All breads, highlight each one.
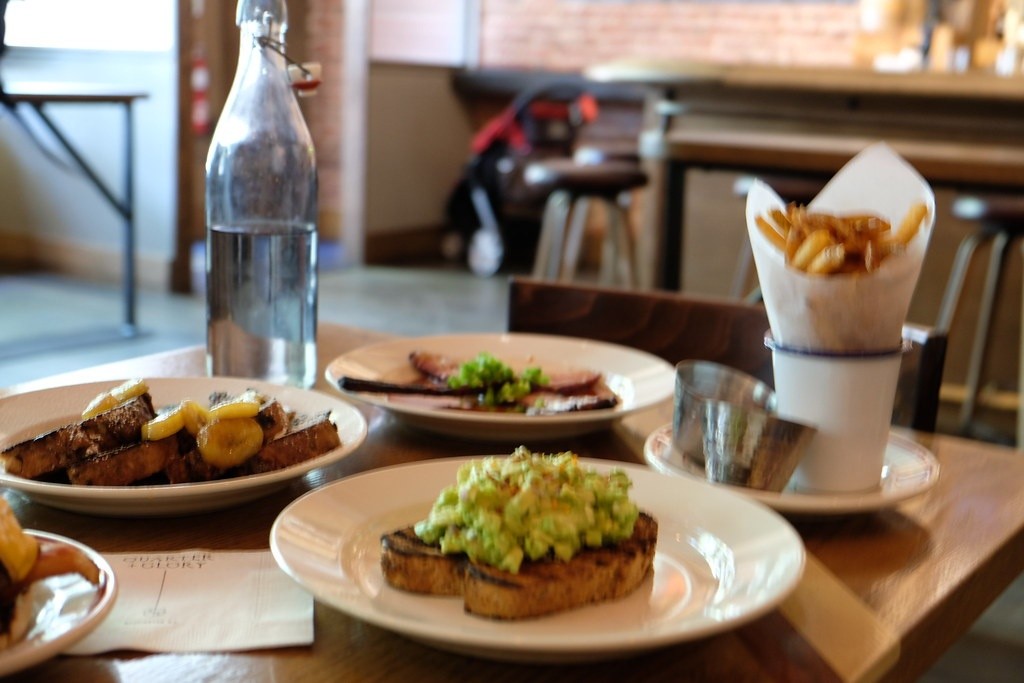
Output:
[0,390,341,485]
[382,511,659,620]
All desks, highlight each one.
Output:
[650,125,1024,294]
[585,56,1024,144]
[0,82,151,339]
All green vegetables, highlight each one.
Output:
[447,353,549,413]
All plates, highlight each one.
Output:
[269,456,806,660]
[0,379,367,513]
[643,421,940,518]
[325,333,676,441]
[0,530,117,677]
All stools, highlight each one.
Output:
[731,174,828,305]
[934,194,1024,437]
[523,158,649,290]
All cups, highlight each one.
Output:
[674,358,774,467]
[701,400,815,494]
[767,332,909,494]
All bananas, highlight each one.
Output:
[82,380,262,466]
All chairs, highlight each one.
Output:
[506,277,950,433]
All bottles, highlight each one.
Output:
[205,0,319,389]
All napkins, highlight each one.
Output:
[60,550,315,655]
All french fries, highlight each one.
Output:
[753,199,944,290]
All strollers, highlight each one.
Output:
[442,71,593,274]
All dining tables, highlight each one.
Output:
[0,322,1024,683]
